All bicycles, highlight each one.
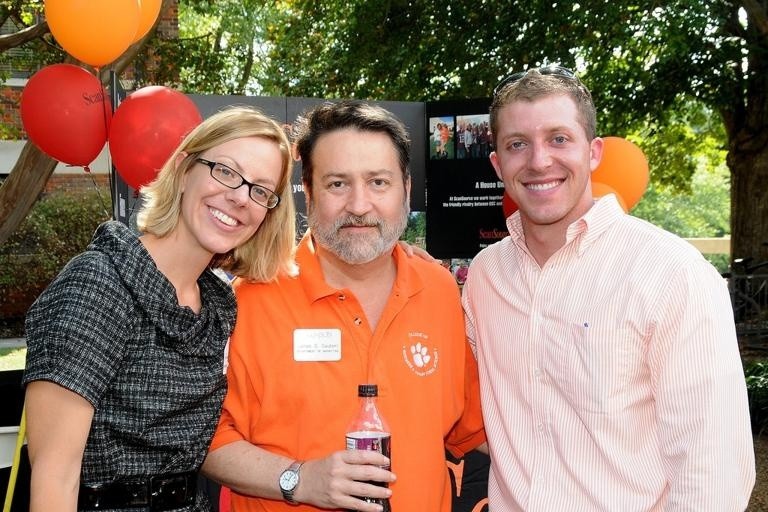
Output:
[720,242,768,324]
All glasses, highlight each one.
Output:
[196,157,279,209]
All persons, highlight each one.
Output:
[433,121,494,160]
[198,101,489,512]
[22,106,300,512]
[462,66,757,512]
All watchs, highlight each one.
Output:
[278,460,306,506]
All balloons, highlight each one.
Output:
[109,85,203,190]
[45,1,140,66]
[20,63,112,171]
[591,136,649,210]
[130,1,161,44]
[592,183,628,214]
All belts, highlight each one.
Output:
[78,470,198,512]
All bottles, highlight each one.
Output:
[343,381,395,510]
